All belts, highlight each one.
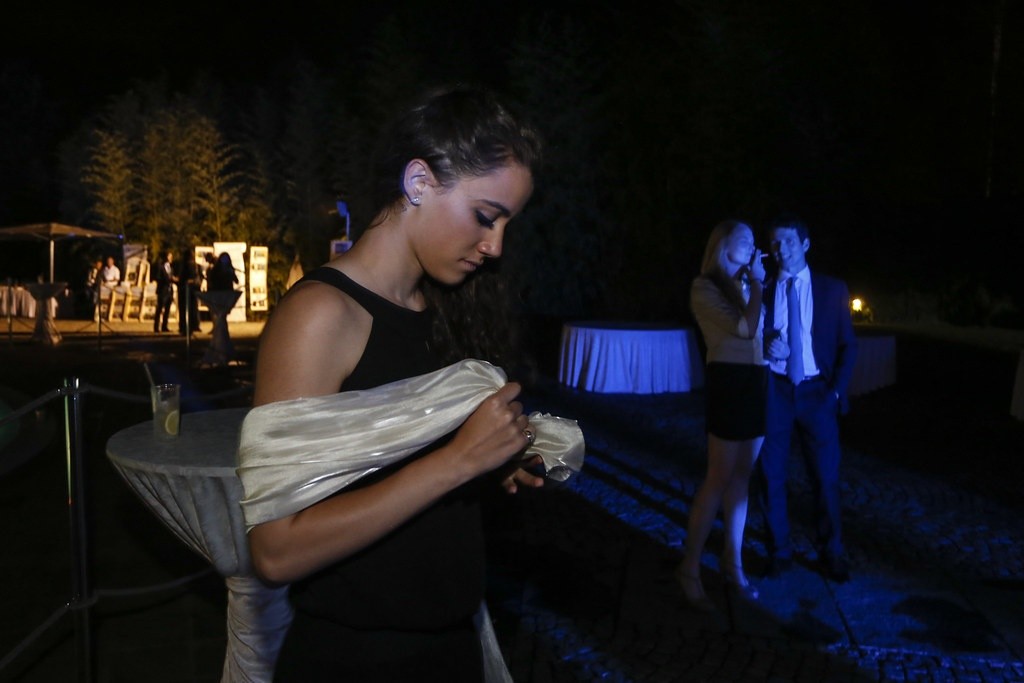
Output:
[772,372,826,383]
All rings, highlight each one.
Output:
[523,429,535,443]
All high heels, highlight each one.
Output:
[720,555,759,601]
[673,566,706,599]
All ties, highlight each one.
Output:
[786,276,806,386]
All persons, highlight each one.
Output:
[669,215,791,614]
[96,256,121,286]
[84,260,103,288]
[758,219,859,583]
[153,249,179,333]
[179,248,240,334]
[237,84,586,683]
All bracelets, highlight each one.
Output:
[750,277,766,288]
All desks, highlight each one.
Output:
[560,320,703,396]
[846,333,898,397]
[106,409,292,683]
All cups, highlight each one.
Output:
[151,383,181,443]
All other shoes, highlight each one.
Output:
[769,554,792,569]
[818,554,850,584]
